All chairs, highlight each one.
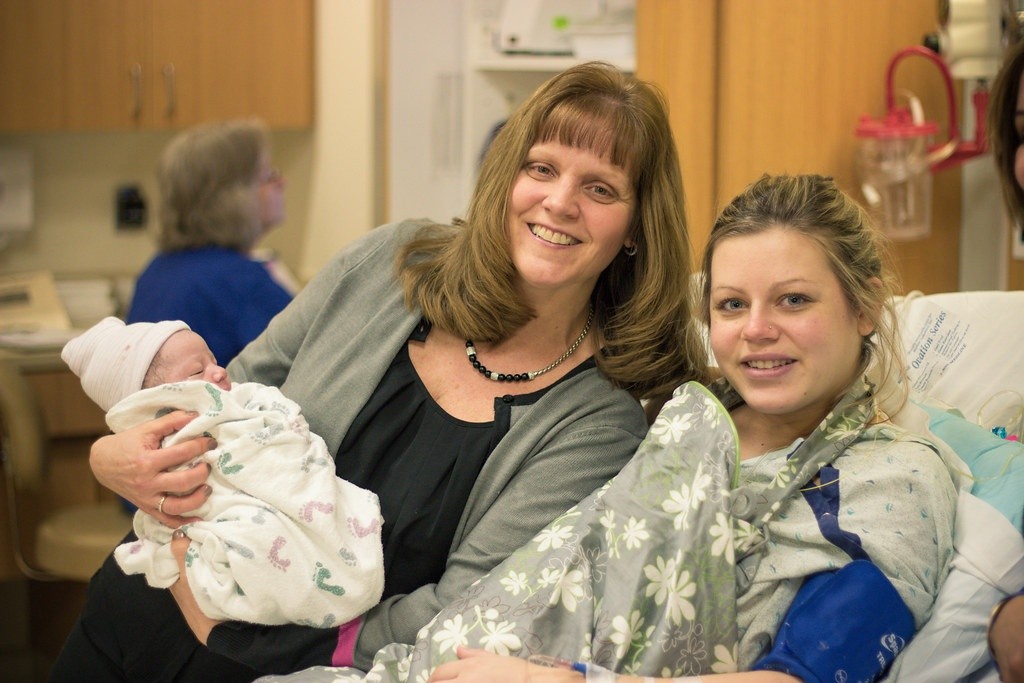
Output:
[0,357,135,581]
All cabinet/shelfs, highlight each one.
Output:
[0,0,315,144]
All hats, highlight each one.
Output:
[62,316,193,414]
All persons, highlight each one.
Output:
[54,61,706,683]
[988,40,1024,683]
[425,173,963,683]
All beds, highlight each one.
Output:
[640,273,1024,445]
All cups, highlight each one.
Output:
[854,106,938,240]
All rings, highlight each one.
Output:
[173,530,186,540]
[157,496,165,515]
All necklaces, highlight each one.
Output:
[462,301,597,383]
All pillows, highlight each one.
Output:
[868,361,1024,683]
